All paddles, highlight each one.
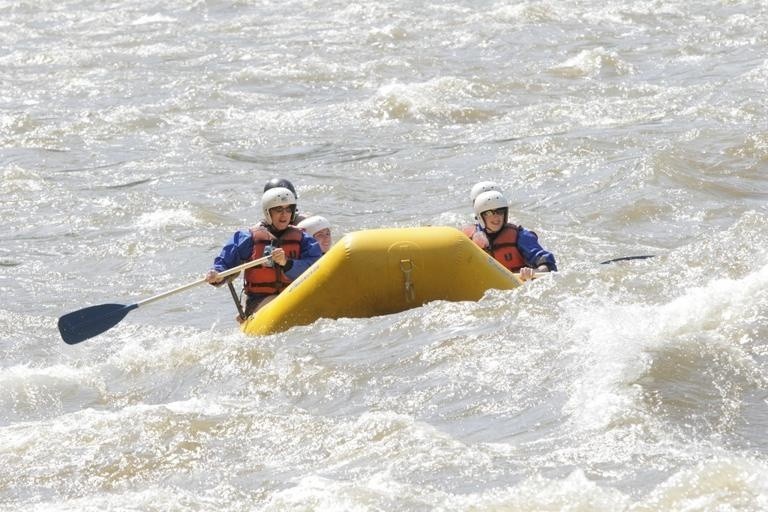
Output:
[57,254,272,344]
[512,255,658,280]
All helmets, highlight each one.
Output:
[261,188,297,225]
[264,178,297,199]
[471,181,502,203]
[474,191,509,229]
[295,216,331,239]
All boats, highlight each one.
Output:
[238,225,524,342]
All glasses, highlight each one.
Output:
[482,208,507,217]
[272,206,293,213]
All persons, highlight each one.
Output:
[206,187,322,317]
[466,191,557,282]
[296,211,332,253]
[462,180,502,238]
[262,178,306,226]
